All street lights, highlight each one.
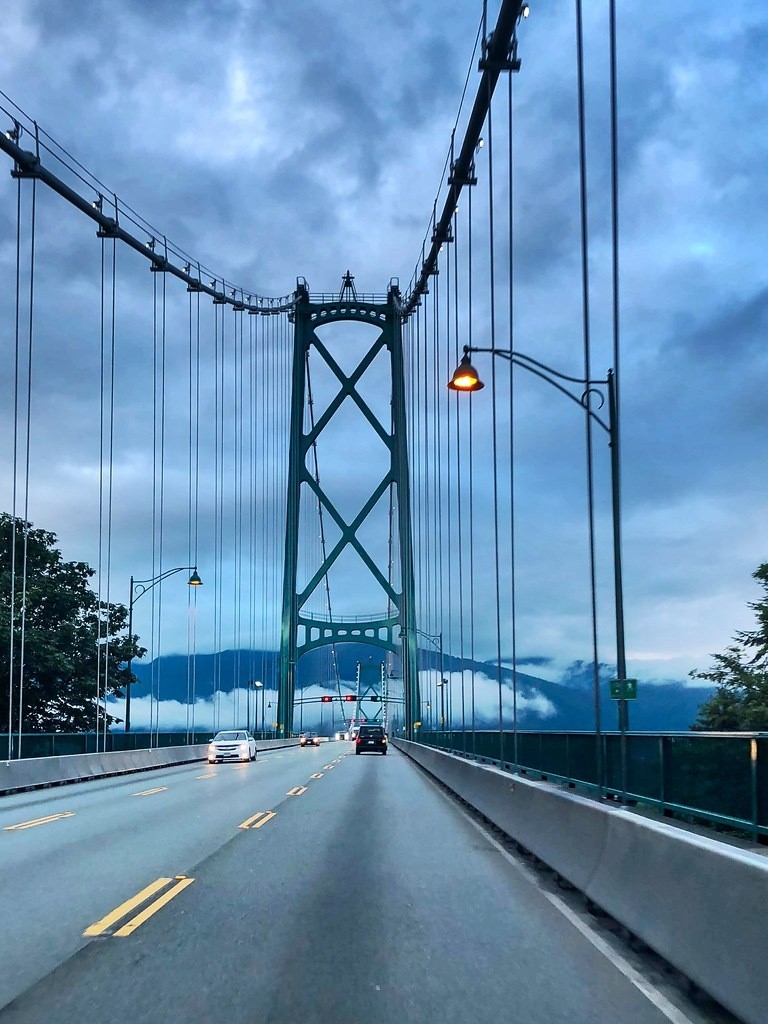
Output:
[446,342,632,807]
[260,653,297,739]
[397,625,446,731]
[123,565,204,731]
[299,681,324,733]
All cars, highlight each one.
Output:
[207,730,258,765]
[299,731,320,746]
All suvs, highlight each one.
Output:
[355,724,388,755]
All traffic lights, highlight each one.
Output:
[371,696,381,701]
[322,697,332,701]
[346,695,356,701]
